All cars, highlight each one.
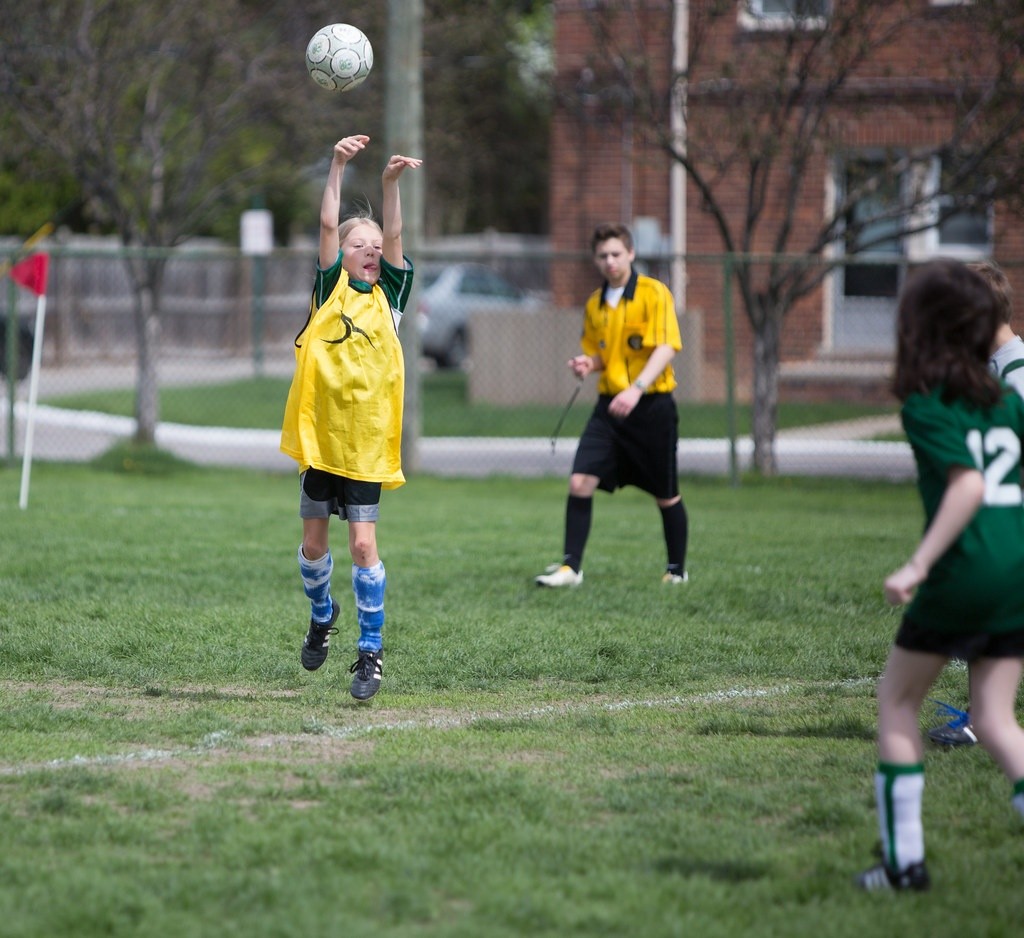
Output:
[407,229,552,373]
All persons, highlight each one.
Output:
[534,223,689,590]
[861,259,1023,896]
[924,263,1023,747]
[279,135,425,702]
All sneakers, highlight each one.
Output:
[535,565,583,587]
[350,647,383,702]
[301,598,340,671]
[926,699,981,745]
[662,570,688,585]
[850,863,928,891]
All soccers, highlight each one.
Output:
[305,23,373,92]
